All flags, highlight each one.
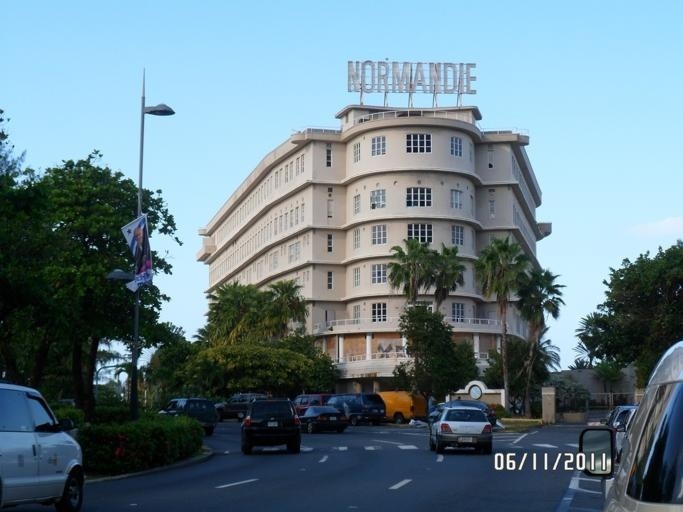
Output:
[118,211,154,291]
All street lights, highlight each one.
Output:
[105,67,175,421]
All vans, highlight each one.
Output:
[0,383,84,512]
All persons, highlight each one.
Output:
[427,391,439,412]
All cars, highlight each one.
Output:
[579,341,683,512]
